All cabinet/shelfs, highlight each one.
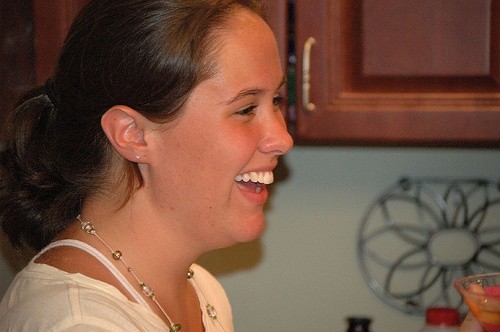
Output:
[33,0,500,149]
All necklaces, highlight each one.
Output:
[75,214,225,332]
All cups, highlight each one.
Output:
[453,273,500,332]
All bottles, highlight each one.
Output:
[420,308,462,332]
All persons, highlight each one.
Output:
[0,0,483,332]
